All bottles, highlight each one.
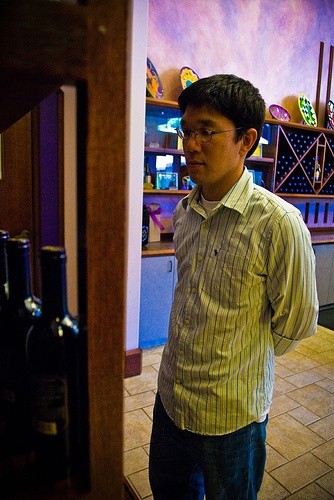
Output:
[275,131,334,198]
[0,231,82,479]
[145,163,151,183]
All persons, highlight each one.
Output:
[148,74,319,500]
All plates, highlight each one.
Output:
[147,58,164,99]
[269,105,291,122]
[298,97,317,128]
[179,66,200,92]
[327,100,334,129]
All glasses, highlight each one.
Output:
[175,126,250,143]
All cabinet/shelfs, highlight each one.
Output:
[139,98,334,349]
